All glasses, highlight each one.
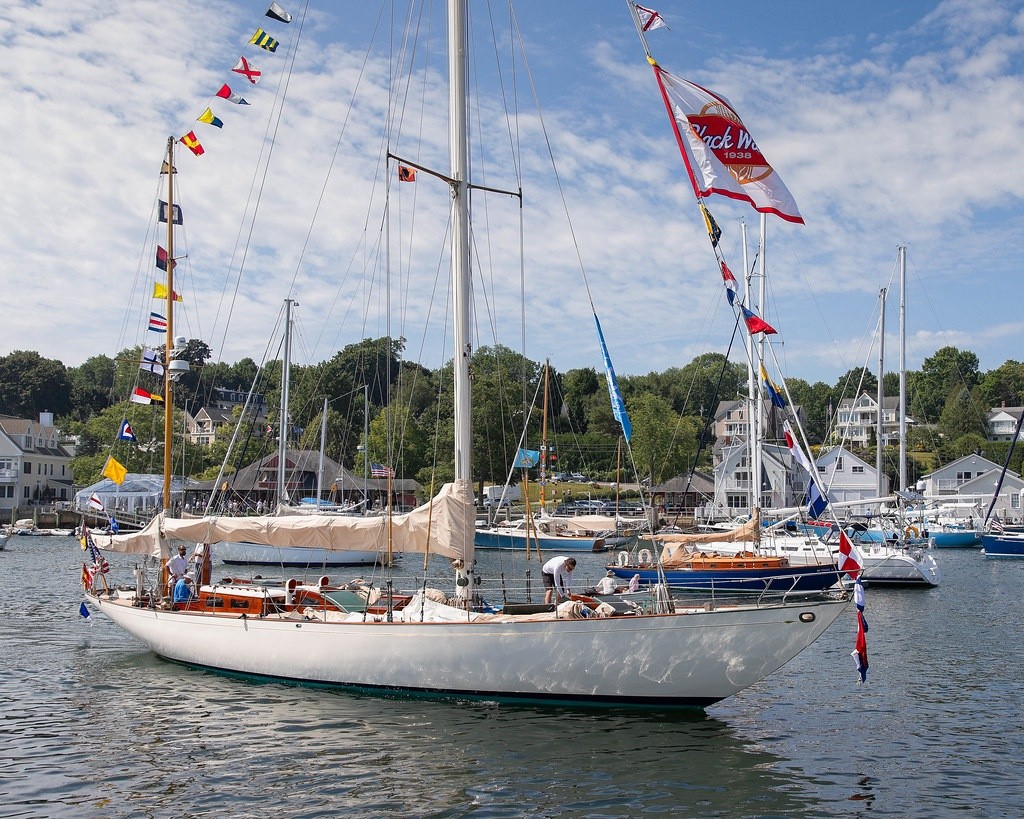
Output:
[567,565,573,571]
[180,549,186,551]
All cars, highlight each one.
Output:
[554,472,575,482]
[484,498,511,510]
[571,473,588,483]
[601,500,642,516]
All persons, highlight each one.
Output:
[173,572,198,602]
[188,542,213,584]
[597,570,620,595]
[905,502,940,523]
[540,555,576,604]
[845,506,853,522]
[865,508,872,528]
[169,494,275,515]
[628,573,648,593]
[164,545,188,598]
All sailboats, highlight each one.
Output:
[75,1,869,717]
[0,245,1024,589]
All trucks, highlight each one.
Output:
[473,483,521,506]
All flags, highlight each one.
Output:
[80,564,92,591]
[636,4,672,32]
[398,166,417,182]
[88,536,110,575]
[699,204,870,682]
[177,1,293,158]
[370,462,394,477]
[538,445,558,504]
[653,66,805,226]
[76,162,186,554]
[991,514,1004,532]
[514,448,541,468]
[594,313,632,443]
[79,603,94,624]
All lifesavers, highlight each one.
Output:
[618,551,628,566]
[906,527,919,538]
[140,521,145,528]
[638,548,652,563]
[571,595,599,609]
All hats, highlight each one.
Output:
[634,574,640,580]
[606,570,615,575]
[183,572,196,582]
